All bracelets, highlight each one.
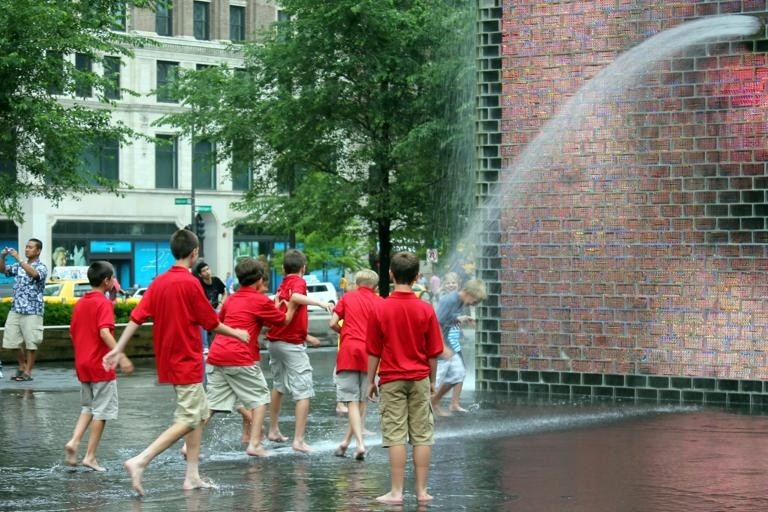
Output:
[218,300,224,305]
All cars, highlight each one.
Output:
[0,279,144,308]
[125,286,148,299]
[373,282,434,301]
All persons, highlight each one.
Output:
[225,270,234,296]
[265,250,331,453]
[181,258,297,456]
[64,260,135,471]
[52,246,67,266]
[73,240,87,266]
[329,254,489,504]
[233,268,270,444]
[0,238,48,381]
[102,230,251,499]
[189,263,227,354]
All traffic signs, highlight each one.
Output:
[195,205,212,213]
[174,197,192,205]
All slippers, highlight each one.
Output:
[10,371,24,380]
[16,373,33,381]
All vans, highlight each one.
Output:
[268,281,339,310]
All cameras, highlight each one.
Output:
[5,248,13,252]
